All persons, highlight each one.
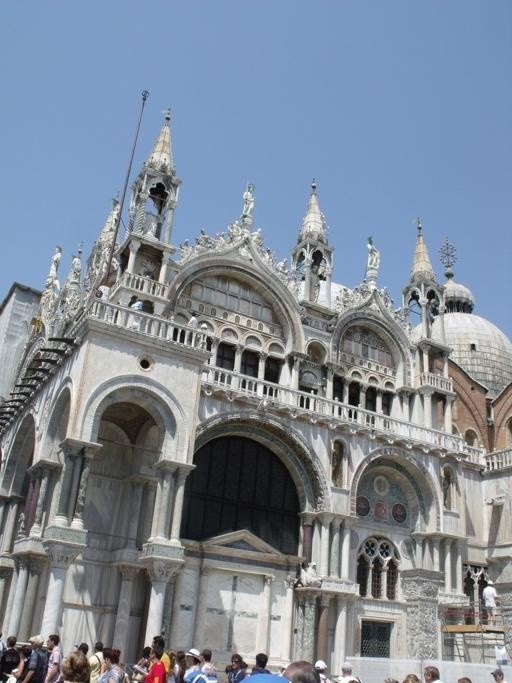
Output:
[187,312,199,345]
[243,184,256,214]
[128,298,143,330]
[384,665,444,683]
[490,670,507,683]
[458,677,472,683]
[482,580,502,625]
[1,631,359,683]
[97,282,112,322]
[366,236,380,270]
[49,246,62,282]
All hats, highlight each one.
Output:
[341,663,353,672]
[490,669,504,676]
[488,581,495,586]
[315,660,328,670]
[185,649,201,663]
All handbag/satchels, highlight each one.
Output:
[20,651,43,682]
